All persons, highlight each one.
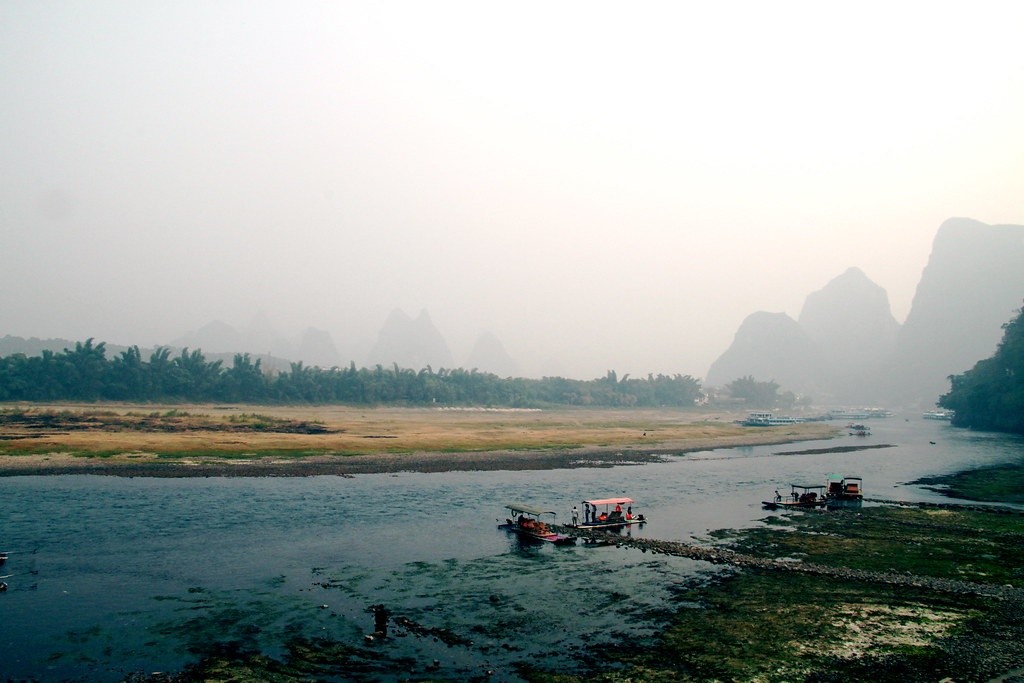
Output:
[373,604,390,638]
[505,504,636,530]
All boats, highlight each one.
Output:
[827,477,863,500]
[762,482,828,507]
[497,502,578,547]
[850,423,873,436]
[563,497,647,532]
